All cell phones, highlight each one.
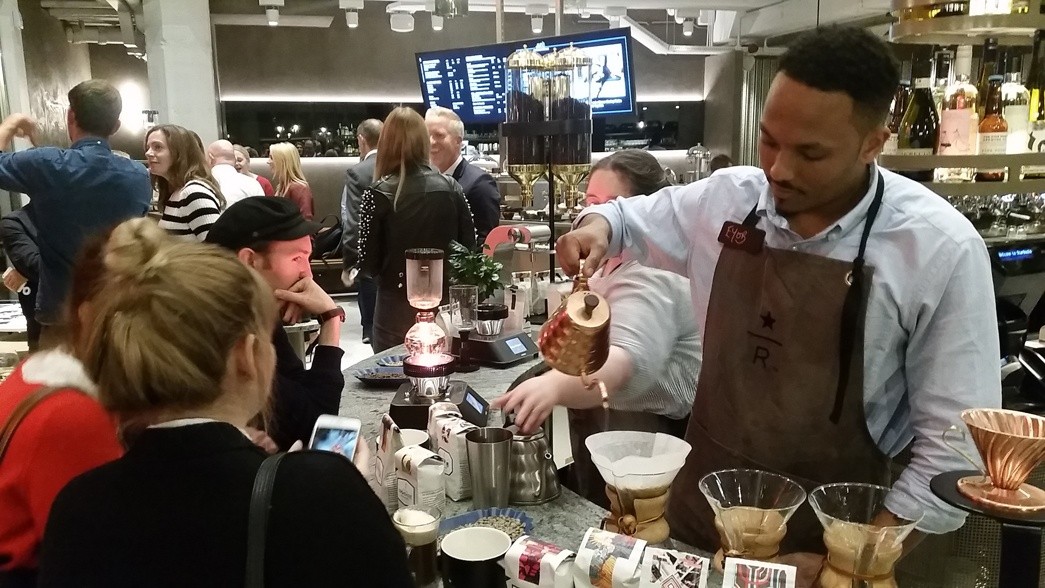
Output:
[306,414,363,463]
[2,267,31,295]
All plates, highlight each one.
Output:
[434,504,533,558]
[351,353,410,385]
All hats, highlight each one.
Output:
[204,197,324,249]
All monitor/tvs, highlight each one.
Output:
[415,25,638,127]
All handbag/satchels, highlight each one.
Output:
[312,213,345,260]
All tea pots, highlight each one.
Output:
[538,259,613,408]
[507,422,562,506]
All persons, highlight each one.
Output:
[489,148,704,509]
[710,154,733,177]
[0,79,502,588]
[552,22,1003,588]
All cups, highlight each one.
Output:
[943,408,1044,515]
[375,430,430,457]
[586,424,691,551]
[448,282,479,331]
[699,468,806,568]
[809,482,926,587]
[466,427,514,511]
[388,500,435,583]
[439,525,513,587]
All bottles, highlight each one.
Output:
[889,27,1043,188]
